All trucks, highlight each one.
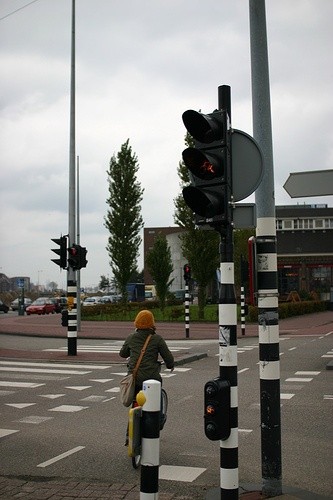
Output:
[125,283,158,303]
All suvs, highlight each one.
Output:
[174,288,185,302]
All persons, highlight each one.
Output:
[118,310,174,448]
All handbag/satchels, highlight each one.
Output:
[119,374,135,407]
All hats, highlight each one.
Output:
[133,310,154,329]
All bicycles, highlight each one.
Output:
[129,361,174,469]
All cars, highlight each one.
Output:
[0,298,67,315]
[81,294,123,307]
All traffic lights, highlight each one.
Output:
[66,241,80,270]
[181,108,227,221]
[184,264,190,281]
